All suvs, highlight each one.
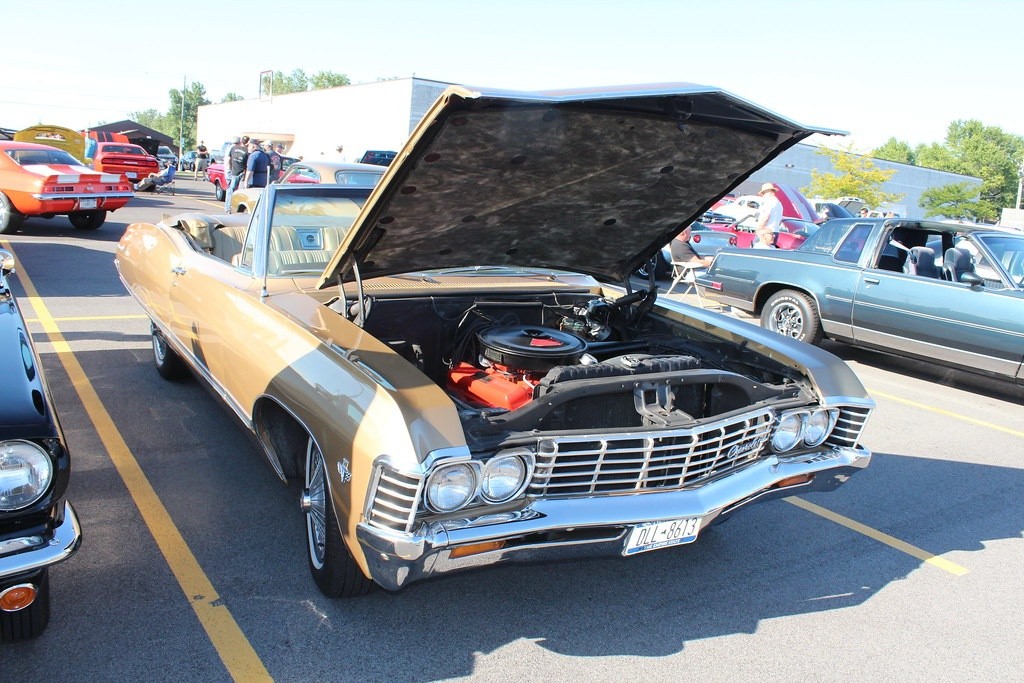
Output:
[355,150,400,188]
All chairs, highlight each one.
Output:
[152,165,176,196]
[231,249,337,274]
[212,226,302,263]
[902,246,940,278]
[321,226,350,250]
[942,248,975,283]
[668,243,708,304]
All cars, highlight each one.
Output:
[231,160,391,253]
[88,131,160,192]
[0,139,137,235]
[206,155,321,202]
[0,249,83,644]
[156,146,179,172]
[693,219,1024,402]
[179,151,211,173]
[113,83,876,600]
[636,181,1024,285]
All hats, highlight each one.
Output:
[261,140,273,147]
[757,183,779,196]
[242,136,249,143]
[277,144,284,149]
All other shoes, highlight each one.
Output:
[134,184,139,190]
[203,179,205,181]
[195,178,197,180]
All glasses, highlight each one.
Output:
[766,232,773,235]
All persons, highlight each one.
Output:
[861,208,894,219]
[755,183,784,248]
[134,159,175,190]
[752,227,776,249]
[671,226,713,281]
[223,136,285,213]
[194,140,207,181]
